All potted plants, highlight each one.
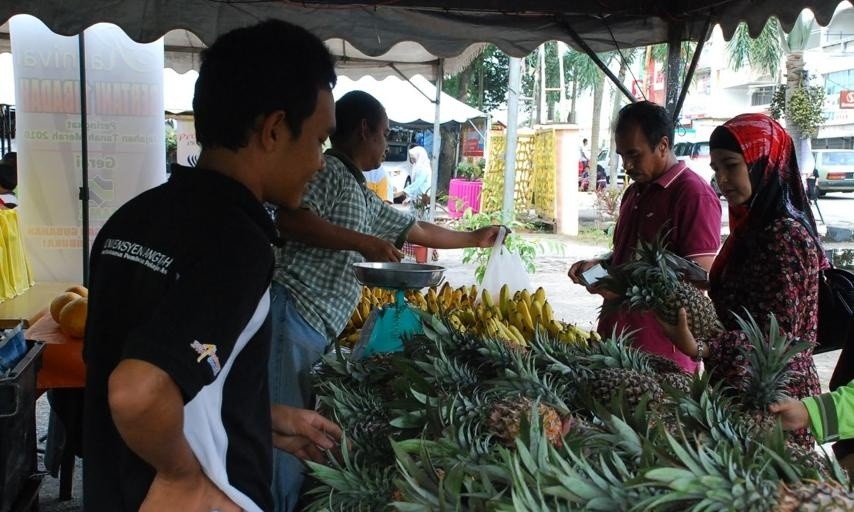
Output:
[588,181,622,232]
[448,158,485,218]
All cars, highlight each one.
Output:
[812,149,854,198]
[597,147,630,184]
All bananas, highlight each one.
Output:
[342,281,601,355]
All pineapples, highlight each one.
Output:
[302,306,853,512]
[595,218,718,341]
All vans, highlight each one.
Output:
[674,139,723,199]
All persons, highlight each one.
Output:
[642,112,829,459]
[579,138,589,169]
[261,87,514,511]
[76,17,355,512]
[765,377,853,447]
[565,100,723,381]
[390,146,432,262]
[805,167,820,198]
[579,164,607,192]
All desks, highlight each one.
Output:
[24,313,86,500]
[0,281,81,329]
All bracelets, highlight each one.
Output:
[687,338,704,362]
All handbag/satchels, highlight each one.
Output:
[795,216,854,355]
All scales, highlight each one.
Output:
[350,261,447,361]
